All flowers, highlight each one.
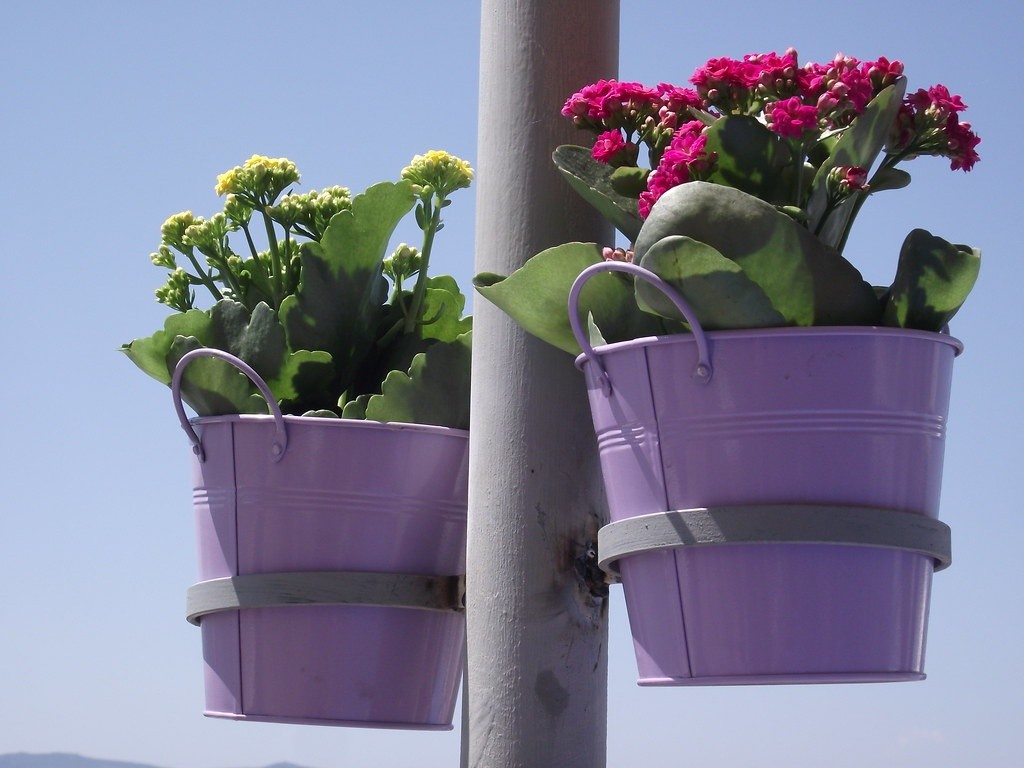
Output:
[473,46,983,352]
[116,147,473,426]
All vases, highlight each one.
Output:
[168,349,470,729]
[568,261,967,687]
